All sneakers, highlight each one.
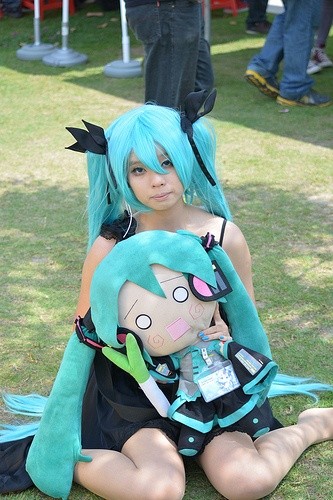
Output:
[244,66,280,99]
[244,19,274,37]
[272,87,332,109]
[306,43,331,75]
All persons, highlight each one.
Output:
[244,0,332,109]
[0,87,332,500]
[245,1,333,73]
[123,0,214,116]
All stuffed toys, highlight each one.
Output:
[77,229,280,455]
